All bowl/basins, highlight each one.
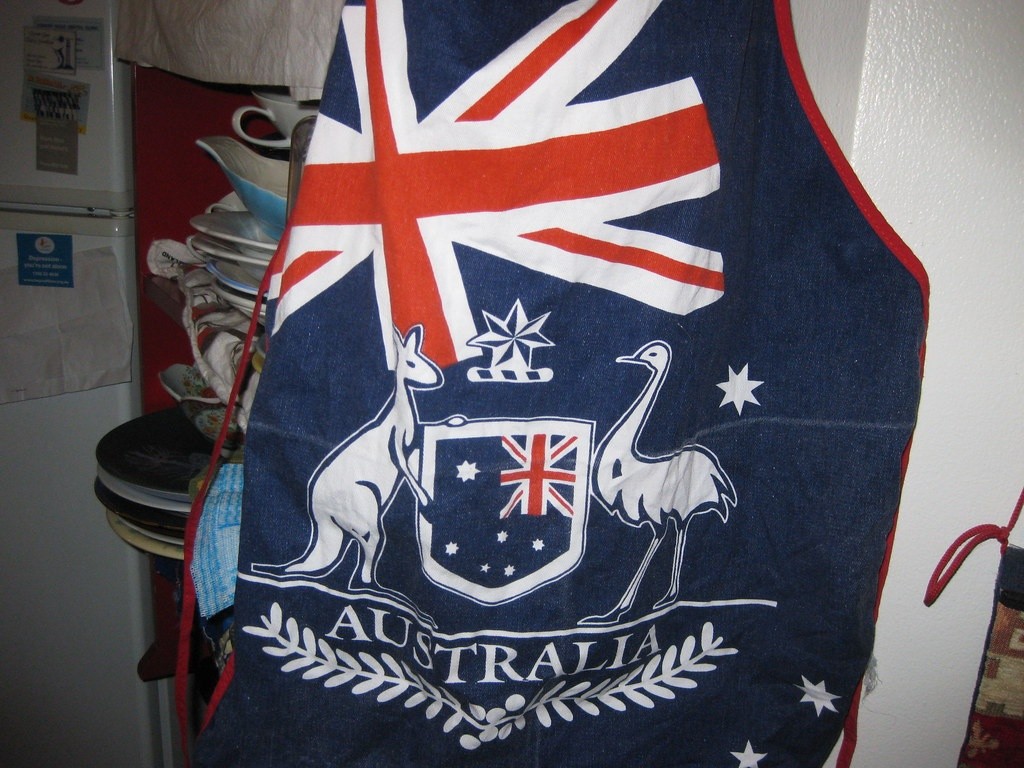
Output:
[232,86,321,149]
[157,360,241,448]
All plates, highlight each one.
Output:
[94,407,231,561]
[187,136,290,324]
